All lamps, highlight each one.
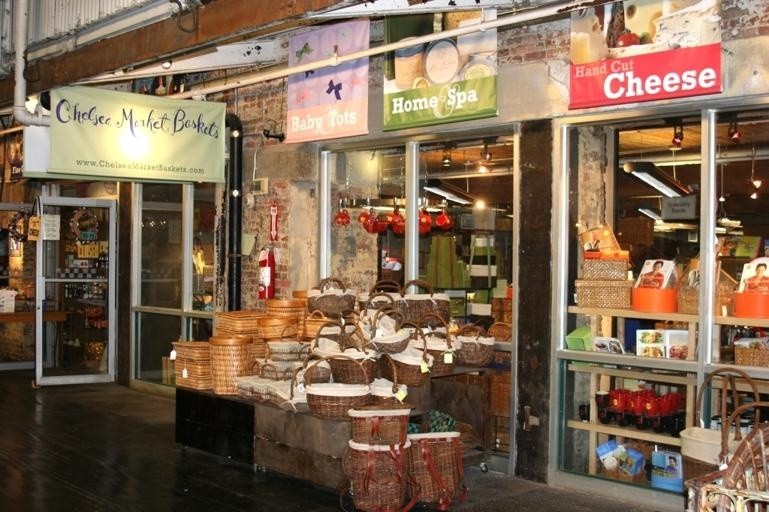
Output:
[480,138,492,161]
[672,117,684,147]
[623,131,693,198]
[441,148,452,167]
[727,111,742,144]
[423,151,478,206]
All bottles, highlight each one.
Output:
[395,37,424,91]
[95,256,109,280]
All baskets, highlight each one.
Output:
[252,276,512,511]
[252,276,512,421]
[339,405,467,512]
[679,367,769,511]
[678,286,722,315]
[575,251,631,308]
[734,337,769,367]
[172,308,264,395]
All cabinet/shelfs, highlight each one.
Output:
[565,305,769,478]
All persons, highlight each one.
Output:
[743,263,769,294]
[638,261,664,289]
[666,456,677,473]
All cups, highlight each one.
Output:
[332,210,454,236]
[578,389,686,438]
[571,32,593,65]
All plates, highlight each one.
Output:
[424,39,461,86]
[606,42,667,58]
